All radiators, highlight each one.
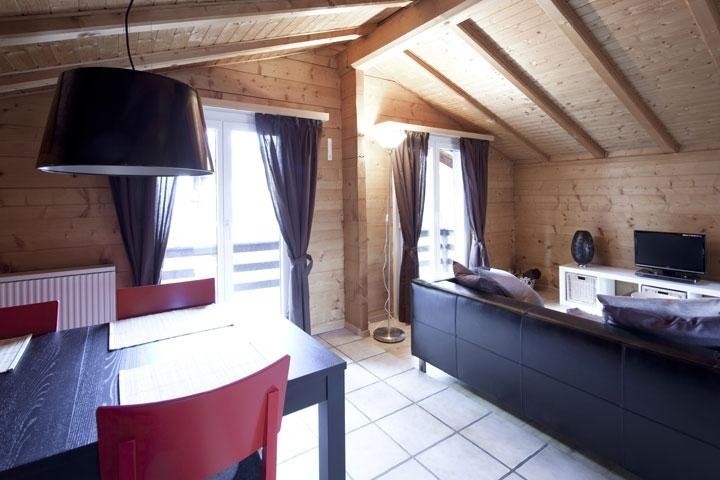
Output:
[0,264,117,322]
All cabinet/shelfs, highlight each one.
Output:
[559,261,720,318]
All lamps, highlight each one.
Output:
[34,0,215,180]
[374,124,408,344]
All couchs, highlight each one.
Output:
[410,270,720,479]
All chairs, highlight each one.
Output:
[117,277,215,321]
[0,301,57,336]
[95,356,289,480]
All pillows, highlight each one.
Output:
[452,260,545,308]
[594,289,720,350]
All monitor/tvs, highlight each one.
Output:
[634,230,706,284]
